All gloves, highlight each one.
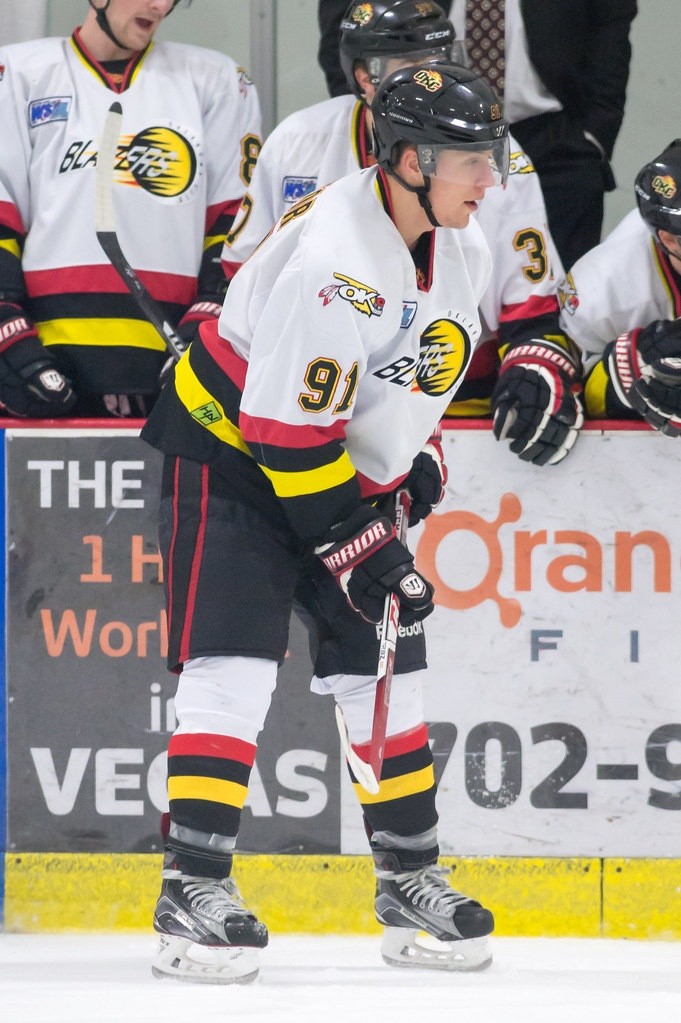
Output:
[603,319,681,437]
[490,340,585,466]
[312,504,435,627]
[162,301,222,387]
[0,298,76,415]
[393,421,449,526]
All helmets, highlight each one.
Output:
[338,1,457,87]
[634,138,681,255]
[371,61,510,186]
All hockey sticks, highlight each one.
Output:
[94,101,187,361]
[334,489,411,796]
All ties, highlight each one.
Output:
[464,0,506,101]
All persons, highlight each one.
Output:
[0,1,264,422]
[560,138,681,439]
[318,0,638,275]
[143,61,501,947]
[220,0,583,466]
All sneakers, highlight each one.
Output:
[148,836,268,985]
[371,850,496,969]
